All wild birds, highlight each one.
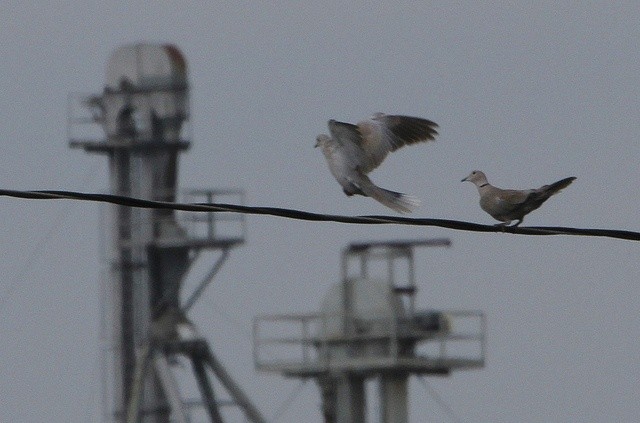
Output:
[312,111,441,215]
[460,169,578,226]
